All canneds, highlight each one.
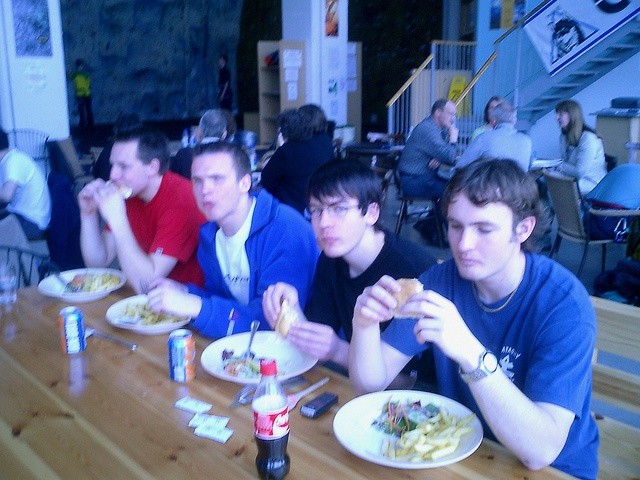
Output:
[167,328,196,382]
[58,306,87,354]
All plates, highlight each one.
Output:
[38,269,127,302]
[333,389,484,469]
[106,294,192,335]
[200,330,318,383]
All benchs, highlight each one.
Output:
[590,296,640,479]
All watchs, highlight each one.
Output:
[458,348,498,384]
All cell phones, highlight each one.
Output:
[299,391,338,419]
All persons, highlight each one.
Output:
[0,126,52,242]
[347,157,600,480]
[542,101,608,250]
[472,97,502,140]
[581,165,640,259]
[397,99,459,247]
[73,59,94,126]
[216,54,233,113]
[94,113,140,181]
[148,141,318,340]
[448,101,532,179]
[76,128,210,296]
[260,104,336,214]
[262,159,439,393]
[172,110,243,178]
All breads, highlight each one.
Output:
[107,180,136,198]
[392,276,423,318]
[274,299,299,336]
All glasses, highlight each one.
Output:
[303,203,364,220]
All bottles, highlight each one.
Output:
[252,358,290,479]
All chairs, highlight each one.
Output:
[541,169,640,280]
[387,150,444,248]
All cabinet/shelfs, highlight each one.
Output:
[257,39,305,145]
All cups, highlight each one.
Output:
[0,265,17,304]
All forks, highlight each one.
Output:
[242,320,260,364]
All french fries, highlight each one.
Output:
[81,273,121,292]
[382,404,476,464]
